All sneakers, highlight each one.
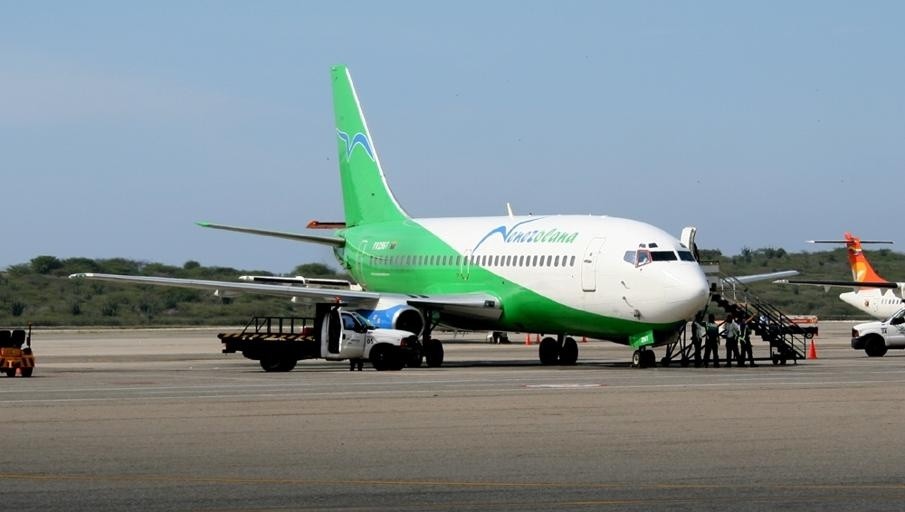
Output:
[723,364,759,367]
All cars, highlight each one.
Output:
[852,301,905,357]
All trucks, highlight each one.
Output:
[755,314,818,341]
[218,304,423,372]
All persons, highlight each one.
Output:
[722,315,740,367]
[704,314,720,368]
[691,314,706,367]
[350,325,362,371]
[739,310,759,367]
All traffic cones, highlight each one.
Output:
[807,338,816,359]
[581,336,587,342]
[526,334,530,345]
[534,333,540,344]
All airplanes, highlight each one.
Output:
[773,231,905,321]
[68,63,800,367]
